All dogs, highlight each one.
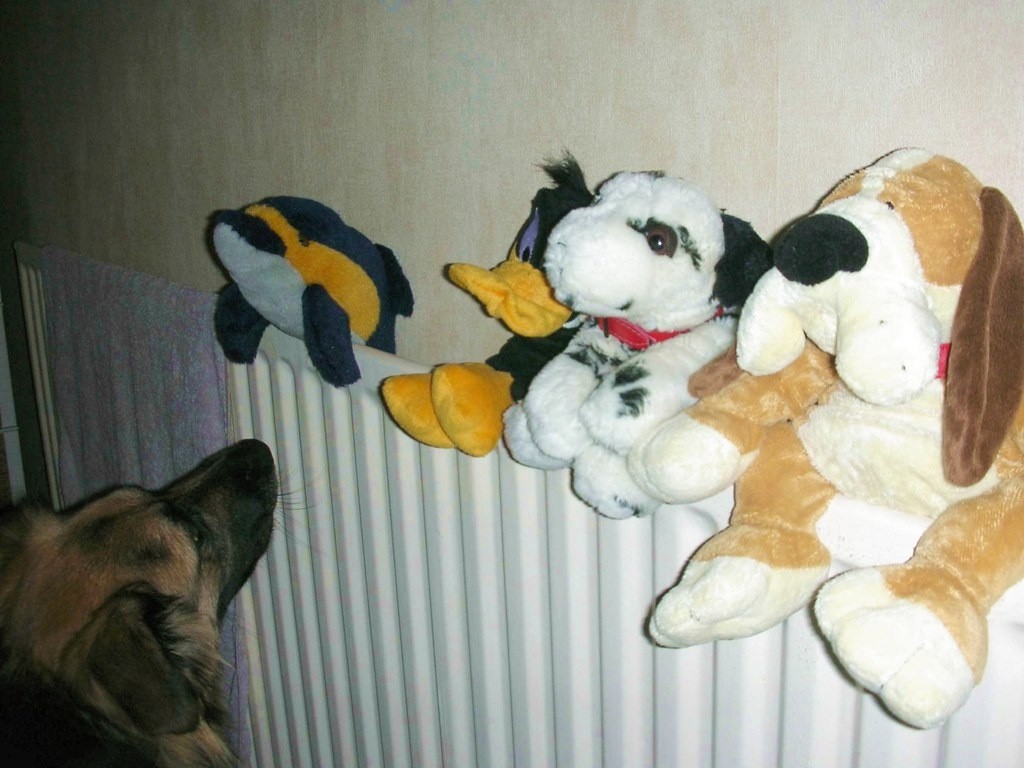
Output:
[1,438,282,767]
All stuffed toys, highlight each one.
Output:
[213,196,414,388]
[503,171,775,519]
[378,156,591,457]
[649,147,1024,728]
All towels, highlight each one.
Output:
[41,244,230,509]
